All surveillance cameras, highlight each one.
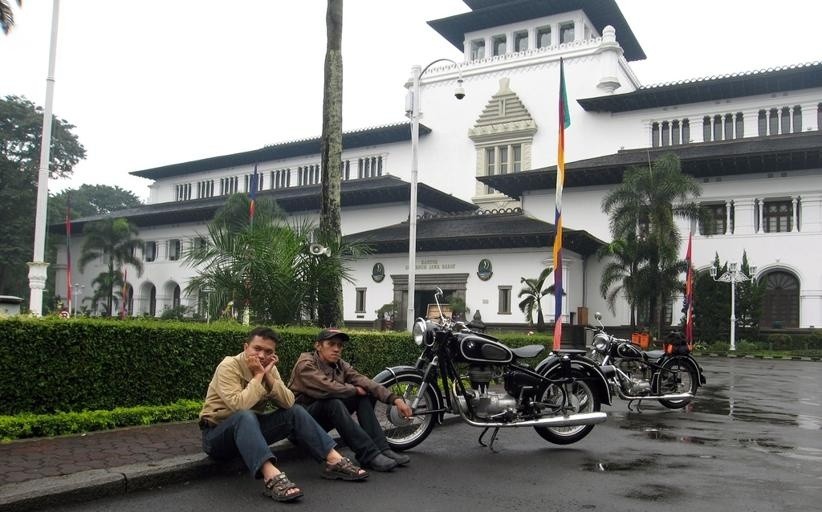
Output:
[455,84,465,100]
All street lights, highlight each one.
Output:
[709,260,759,351]
[404,58,466,332]
[72,283,85,318]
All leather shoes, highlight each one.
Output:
[368,449,410,471]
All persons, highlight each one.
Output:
[199,327,368,502]
[286,327,413,471]
[383,310,395,331]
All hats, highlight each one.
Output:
[316,328,349,340]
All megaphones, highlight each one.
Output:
[311,245,324,255]
[324,248,332,257]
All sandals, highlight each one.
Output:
[263,473,303,501]
[321,457,368,481]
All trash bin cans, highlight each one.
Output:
[632,332,650,350]
